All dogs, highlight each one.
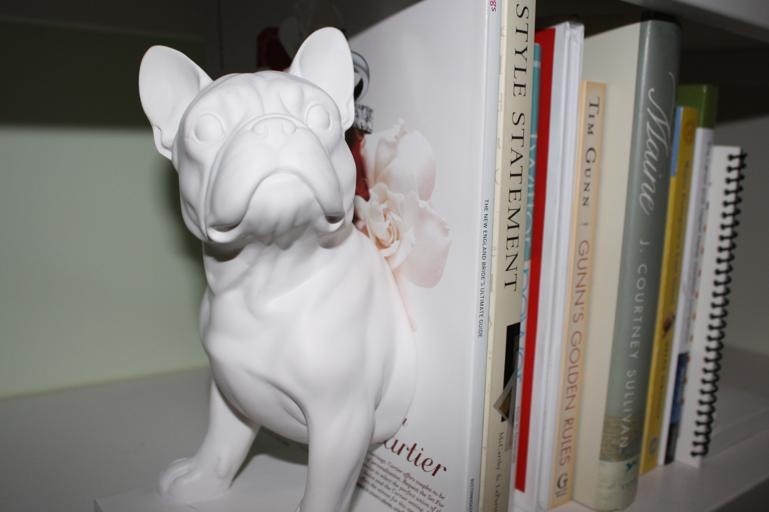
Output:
[138,27,417,512]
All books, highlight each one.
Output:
[348,0,747,512]
